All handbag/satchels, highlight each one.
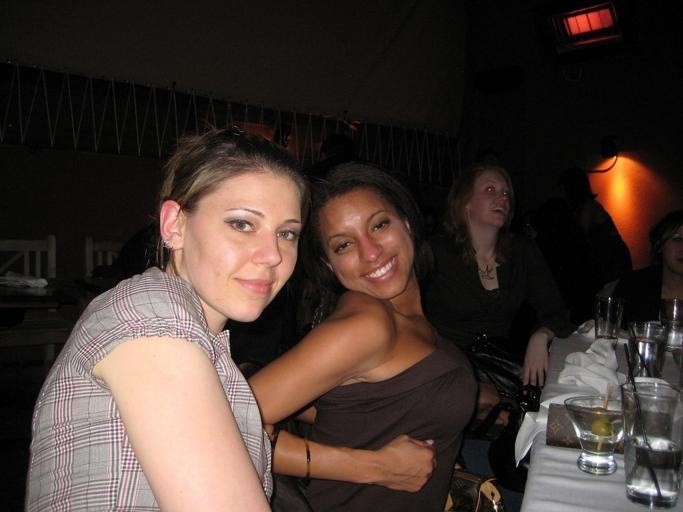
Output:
[442,465,503,511]
[461,331,541,416]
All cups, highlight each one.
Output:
[628,319,669,372]
[658,295,683,351]
[593,296,625,342]
[627,335,659,382]
[621,379,683,509]
[563,395,625,477]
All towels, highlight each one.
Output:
[0,269,49,288]
[513,319,628,467]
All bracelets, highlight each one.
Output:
[300,435,312,482]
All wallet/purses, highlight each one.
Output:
[545,403,670,458]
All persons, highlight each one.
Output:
[411,160,570,511]
[22,124,313,511]
[240,160,479,510]
[599,200,681,333]
[236,359,317,511]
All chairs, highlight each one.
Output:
[0,232,125,366]
